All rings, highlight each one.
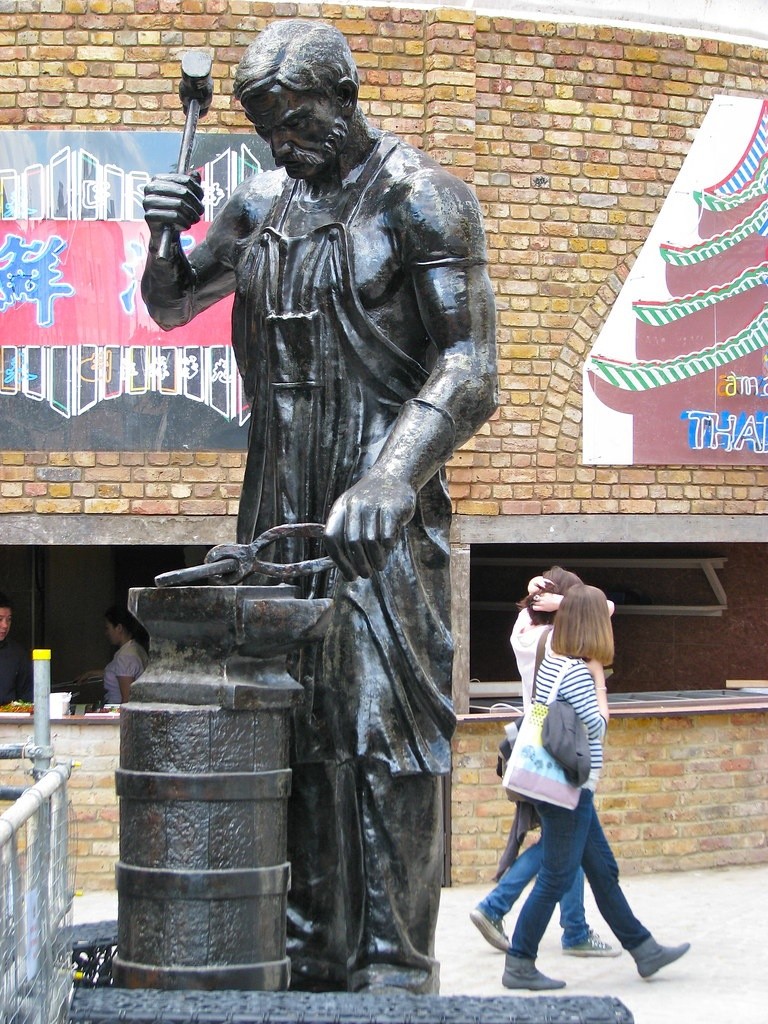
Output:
[595,687,607,691]
[533,594,540,601]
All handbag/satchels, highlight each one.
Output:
[503,659,583,810]
[495,716,526,776]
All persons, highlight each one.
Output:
[74,600,149,705]
[0,593,36,706]
[139,17,502,997]
[468,566,691,991]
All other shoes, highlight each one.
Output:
[502,952,566,989]
[629,936,690,980]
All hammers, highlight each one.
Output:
[155,47,214,269]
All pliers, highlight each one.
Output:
[205,524,338,586]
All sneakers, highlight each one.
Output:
[564,934,622,957]
[471,909,511,951]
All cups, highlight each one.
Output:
[49,694,63,719]
